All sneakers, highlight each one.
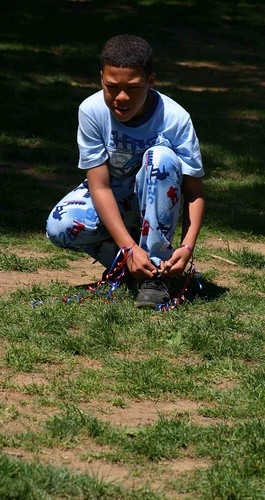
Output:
[135,279,170,308]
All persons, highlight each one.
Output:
[45,33,206,308]
